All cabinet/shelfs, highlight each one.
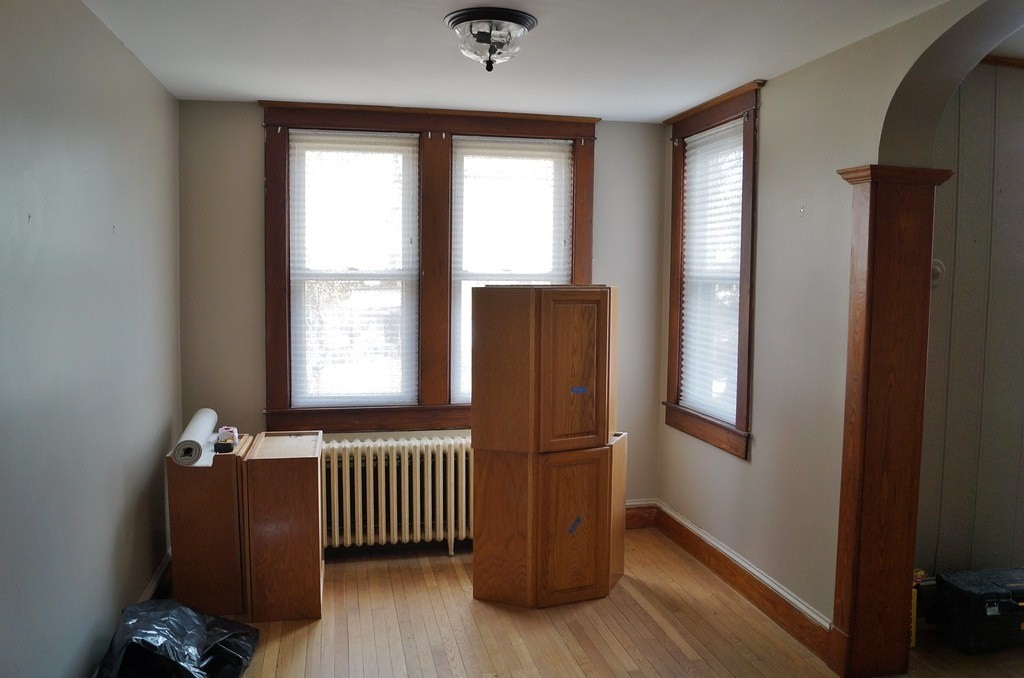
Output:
[471,284,618,455]
[473,432,628,609]
[166,430,326,623]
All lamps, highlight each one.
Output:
[443,6,538,71]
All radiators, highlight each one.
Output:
[320,436,474,557]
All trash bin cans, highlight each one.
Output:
[939,569,1013,654]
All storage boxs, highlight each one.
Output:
[910,569,1024,657]
